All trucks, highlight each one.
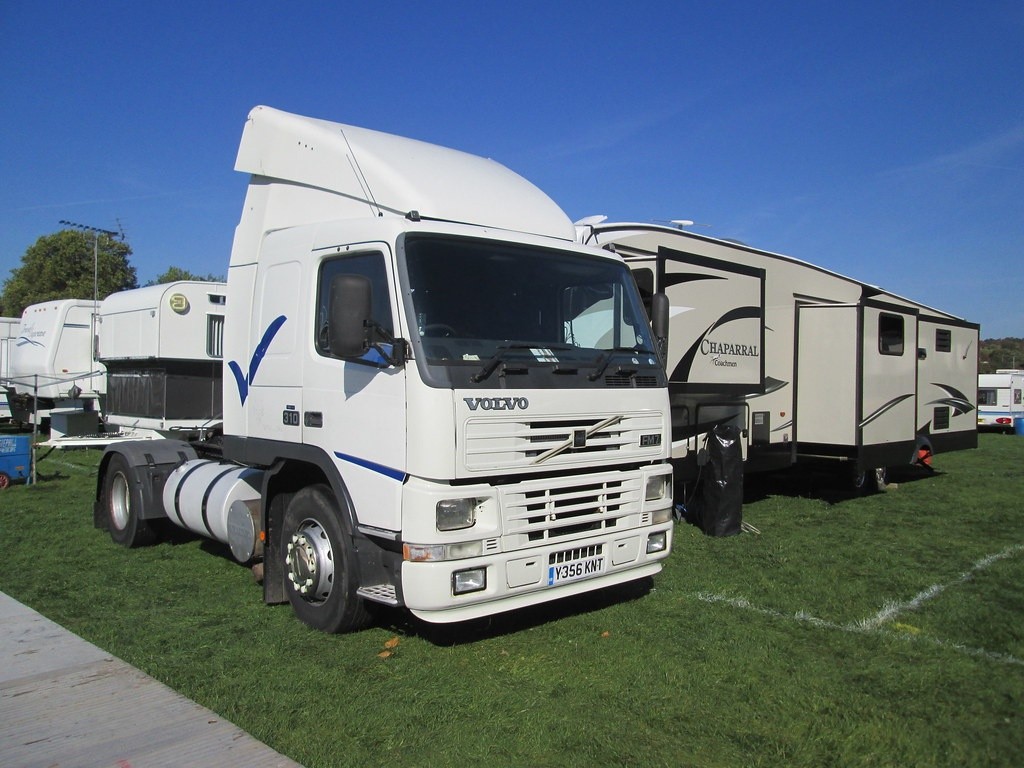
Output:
[575,219,981,494]
[88,103,678,636]
